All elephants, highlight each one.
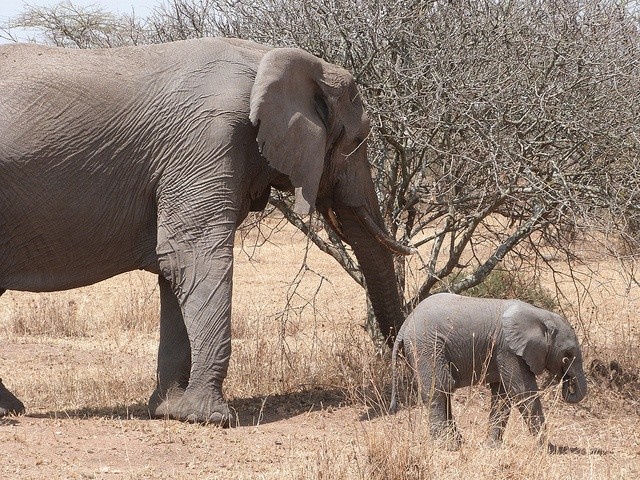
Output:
[388,291,588,450]
[0,37,418,426]
